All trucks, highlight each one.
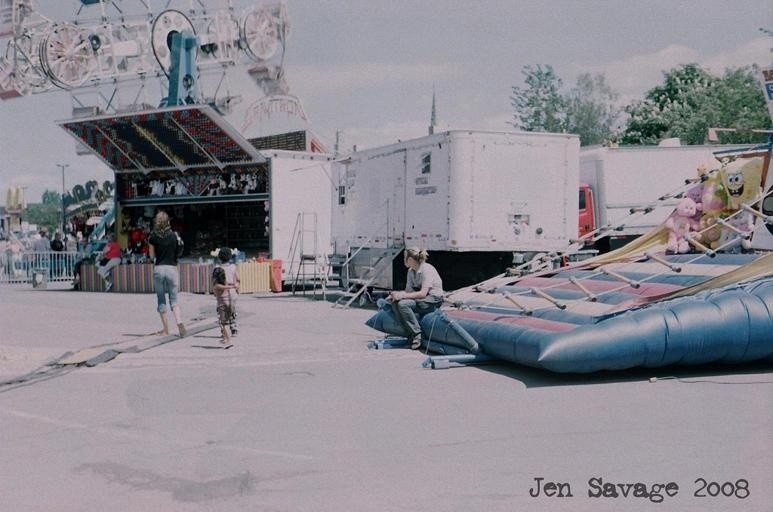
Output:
[510,141,769,279]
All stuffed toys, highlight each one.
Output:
[664,155,766,257]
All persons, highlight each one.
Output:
[146,211,187,339]
[49,233,64,282]
[3,236,11,275]
[121,175,270,265]
[32,230,50,268]
[211,246,240,338]
[383,246,443,350]
[96,232,122,292]
[2,238,23,278]
[211,266,239,351]
[70,233,94,285]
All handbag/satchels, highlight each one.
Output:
[100,258,108,266]
[175,231,184,259]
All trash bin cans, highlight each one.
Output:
[33,268,48,289]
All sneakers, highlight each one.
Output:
[224,344,233,349]
[232,332,238,336]
[152,330,167,337]
[105,283,112,291]
[412,340,420,349]
[177,323,186,337]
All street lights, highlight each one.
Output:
[56,163,68,251]
